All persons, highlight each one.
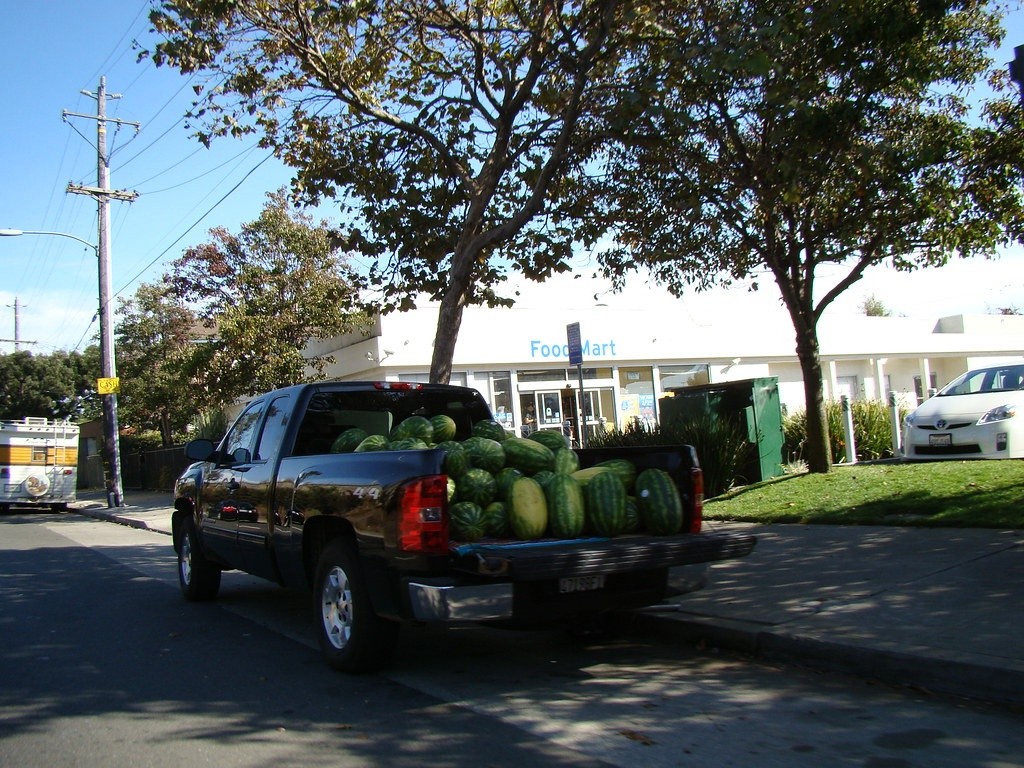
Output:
[523,402,538,438]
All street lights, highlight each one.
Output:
[0,228,122,510]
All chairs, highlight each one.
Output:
[1002,373,1020,388]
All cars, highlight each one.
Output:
[220,503,259,523]
[902,363,1024,459]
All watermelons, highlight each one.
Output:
[333,413,682,537]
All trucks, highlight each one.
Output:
[0,417,80,512]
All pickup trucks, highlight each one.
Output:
[172,380,757,676]
